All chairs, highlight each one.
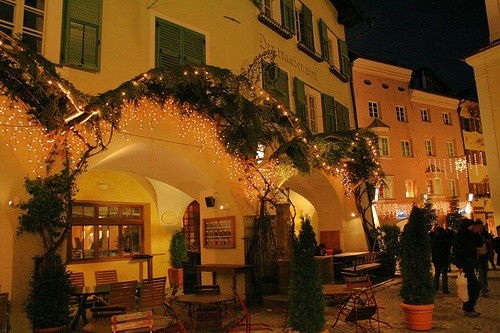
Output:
[335,275,394,333]
[66,269,254,333]
[325,249,352,274]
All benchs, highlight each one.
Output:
[344,253,383,275]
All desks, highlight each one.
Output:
[333,251,370,272]
[70,281,148,329]
[318,284,353,297]
[175,294,234,323]
[195,263,256,296]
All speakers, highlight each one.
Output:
[205,196,214,207]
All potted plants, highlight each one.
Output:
[399,202,441,331]
[22,254,77,333]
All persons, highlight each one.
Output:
[315,242,328,256]
[428,219,458,294]
[74,237,82,249]
[454,218,500,318]
[325,248,342,255]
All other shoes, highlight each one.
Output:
[465,308,481,318]
[442,291,450,294]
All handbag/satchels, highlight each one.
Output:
[457,271,470,303]
